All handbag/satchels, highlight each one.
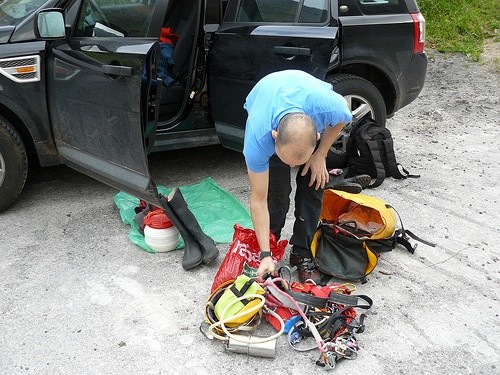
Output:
[211,224,288,294]
[319,189,396,239]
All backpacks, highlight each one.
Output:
[347,117,420,189]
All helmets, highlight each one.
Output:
[204,279,258,334]
[310,228,377,279]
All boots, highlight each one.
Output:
[167,187,220,266]
[158,193,202,270]
[326,167,362,194]
[346,175,370,188]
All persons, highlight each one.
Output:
[242,69,352,286]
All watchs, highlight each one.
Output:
[259,250,273,259]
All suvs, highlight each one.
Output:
[0,0,427,219]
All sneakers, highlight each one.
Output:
[263,272,283,289]
[290,247,324,283]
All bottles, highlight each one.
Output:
[144,209,181,253]
[227,334,278,358]
